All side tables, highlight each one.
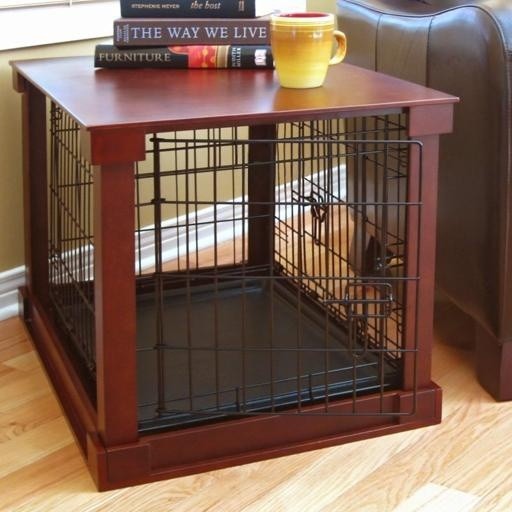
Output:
[8,53,461,492]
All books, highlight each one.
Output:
[93,0,276,71]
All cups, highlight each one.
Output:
[272,11,347,89]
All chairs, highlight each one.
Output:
[335,1,511,404]
